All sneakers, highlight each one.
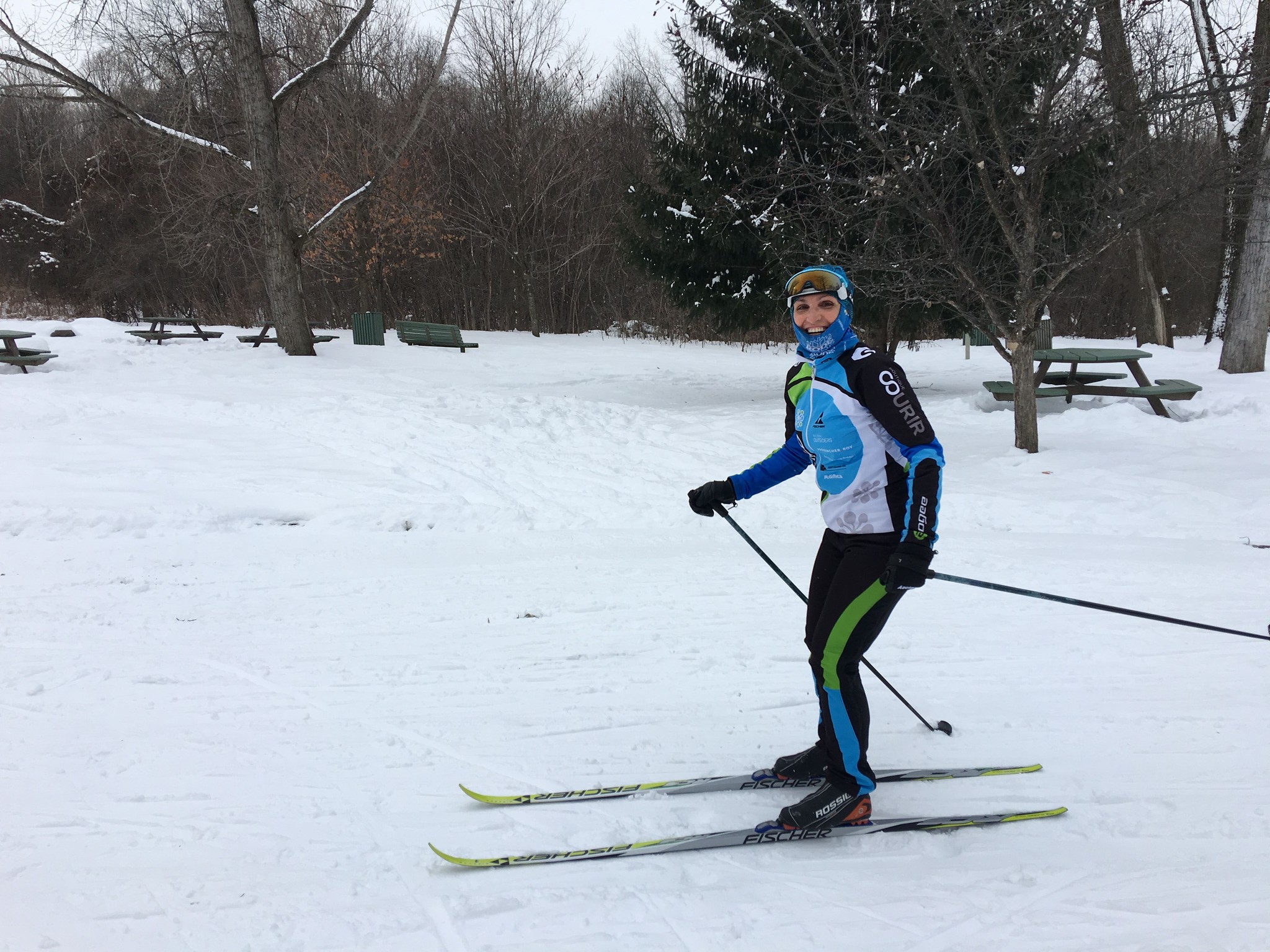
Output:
[773,742,826,779]
[779,778,872,830]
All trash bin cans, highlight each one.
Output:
[351,312,385,346]
[963,321,996,347]
[1033,318,1052,350]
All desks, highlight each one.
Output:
[992,347,1198,420]
[239,321,334,347]
[0,329,50,374]
[132,316,222,345]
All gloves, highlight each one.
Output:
[686,477,736,517]
[878,543,935,593]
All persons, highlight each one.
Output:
[689,265,946,831]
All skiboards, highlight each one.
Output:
[430,764,1070,867]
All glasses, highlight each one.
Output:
[785,269,841,296]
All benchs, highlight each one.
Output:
[0,347,59,361]
[394,319,479,354]
[982,380,1013,394]
[1033,371,1128,380]
[124,330,224,337]
[311,336,333,342]
[235,335,270,339]
[320,335,340,339]
[1133,379,1202,395]
